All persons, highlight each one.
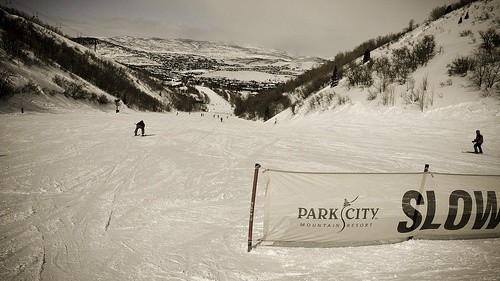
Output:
[135,121,145,136]
[472,130,483,155]
